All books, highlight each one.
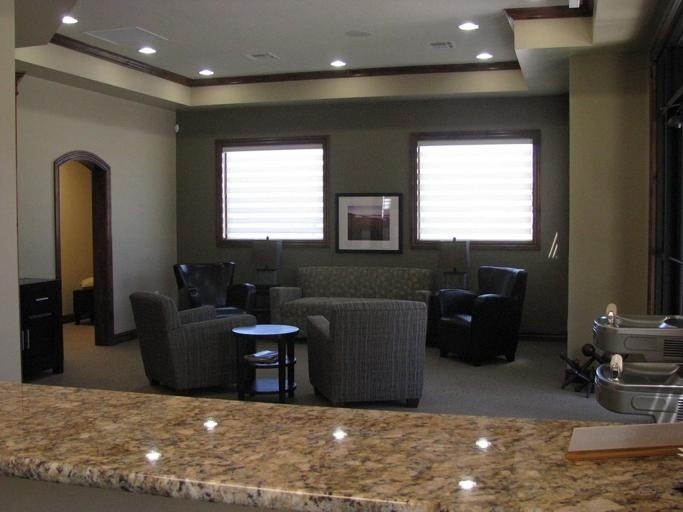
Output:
[566,420,683,461]
[243,348,277,363]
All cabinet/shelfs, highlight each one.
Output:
[17,277,63,385]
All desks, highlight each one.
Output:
[1,382,681,511]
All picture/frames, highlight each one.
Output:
[334,193,403,254]
